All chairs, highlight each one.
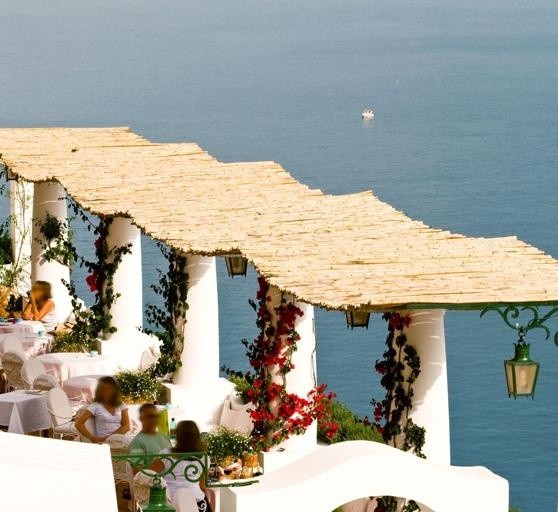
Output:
[0,305,256,512]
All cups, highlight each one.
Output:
[0,318,23,325]
[39,330,45,336]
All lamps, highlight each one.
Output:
[502,326,541,400]
[339,310,370,332]
[224,256,247,279]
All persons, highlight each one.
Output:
[147,421,215,512]
[127,403,173,479]
[75,376,130,444]
[22,281,57,343]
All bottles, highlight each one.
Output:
[169,417,176,437]
